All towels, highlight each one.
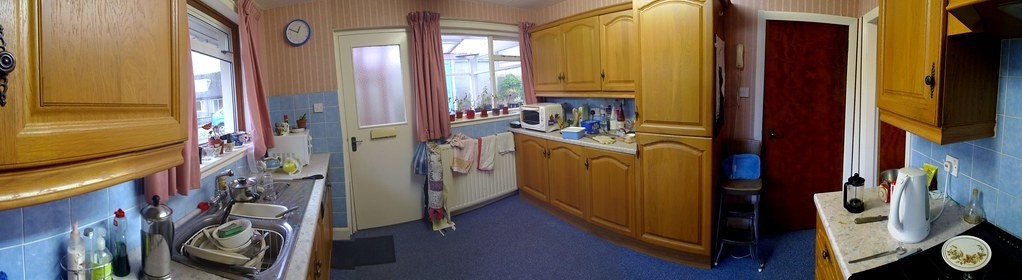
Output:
[445,132,474,176]
[476,134,496,171]
[496,132,515,155]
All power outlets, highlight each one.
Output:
[591,108,599,114]
[945,155,960,177]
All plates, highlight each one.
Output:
[941,236,992,272]
[181,244,250,265]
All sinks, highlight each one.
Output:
[175,215,286,275]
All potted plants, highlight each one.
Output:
[295,112,307,129]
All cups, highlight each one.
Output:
[206,131,249,158]
[962,189,984,225]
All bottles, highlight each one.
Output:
[599,100,628,132]
[111,208,132,278]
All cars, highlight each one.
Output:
[211,107,225,136]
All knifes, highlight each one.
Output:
[854,215,887,223]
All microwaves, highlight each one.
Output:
[519,103,563,132]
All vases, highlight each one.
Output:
[503,107,508,114]
[481,109,487,117]
[450,113,455,121]
[492,108,500,114]
[457,111,463,119]
[467,110,475,118]
[207,137,225,154]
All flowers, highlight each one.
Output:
[448,88,518,112]
[203,123,224,139]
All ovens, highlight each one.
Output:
[265,128,313,167]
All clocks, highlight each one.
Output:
[286,19,310,46]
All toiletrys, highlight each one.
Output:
[90,226,113,280]
[66,219,85,280]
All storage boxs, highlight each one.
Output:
[561,126,586,139]
[582,120,600,133]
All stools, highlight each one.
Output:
[712,139,767,272]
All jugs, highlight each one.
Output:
[843,172,866,213]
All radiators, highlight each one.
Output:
[433,133,518,216]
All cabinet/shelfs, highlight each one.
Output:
[511,134,587,219]
[0,0,191,207]
[633,0,717,134]
[584,149,637,237]
[603,10,638,93]
[636,129,716,256]
[532,14,603,92]
[812,213,845,280]
[876,0,1004,145]
[306,185,334,280]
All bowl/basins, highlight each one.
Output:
[210,220,254,249]
[189,218,266,267]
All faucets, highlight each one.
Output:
[209,167,234,207]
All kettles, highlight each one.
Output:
[887,165,930,243]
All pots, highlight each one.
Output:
[227,177,259,201]
